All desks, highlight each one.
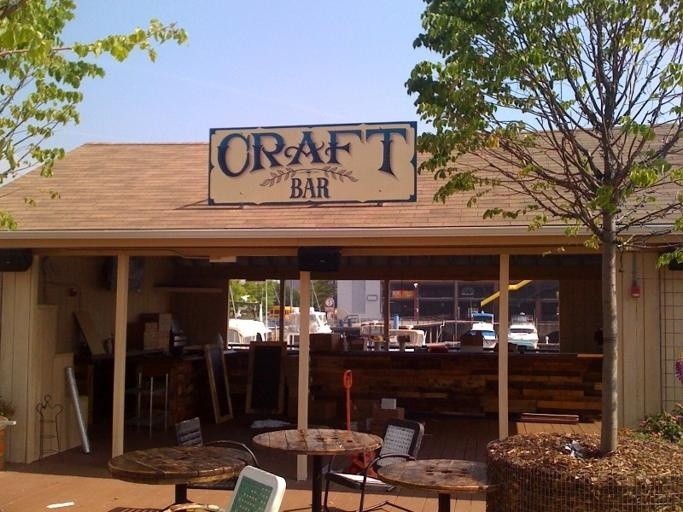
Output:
[379,459,489,512]
[252,429,383,511]
[107,446,252,511]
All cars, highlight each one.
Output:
[507,322,538,351]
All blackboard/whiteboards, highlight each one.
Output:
[204,343,234,424]
[245,340,287,415]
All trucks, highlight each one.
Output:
[471,313,494,336]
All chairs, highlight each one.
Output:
[160,464,286,512]
[324,417,424,511]
[176,417,260,490]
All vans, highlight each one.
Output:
[265,306,293,322]
